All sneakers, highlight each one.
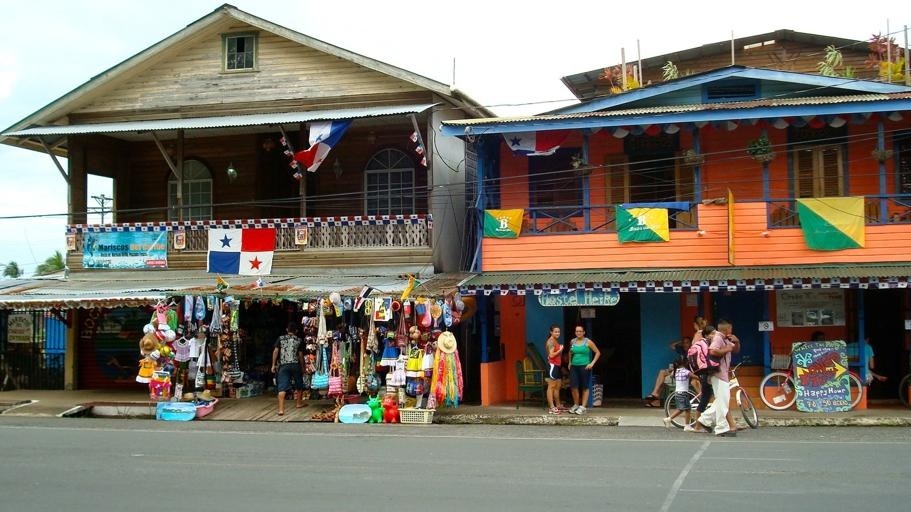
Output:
[683,425,695,432]
[547,407,561,415]
[569,403,587,415]
[556,405,568,413]
[662,418,671,430]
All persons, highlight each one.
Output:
[542,323,569,416]
[567,326,601,414]
[269,322,311,417]
[849,331,888,387]
[641,315,746,439]
[809,330,826,341]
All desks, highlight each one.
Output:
[665,383,694,408]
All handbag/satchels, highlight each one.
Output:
[225,365,241,379]
[193,368,218,390]
[309,331,430,396]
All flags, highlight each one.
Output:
[293,115,354,175]
[204,225,278,276]
[615,205,669,243]
[794,196,865,252]
[483,207,524,239]
[499,129,564,159]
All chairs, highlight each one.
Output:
[514,343,569,411]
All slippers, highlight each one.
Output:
[695,429,709,434]
[644,402,661,408]
[645,394,658,401]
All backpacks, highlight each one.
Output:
[686,339,720,376]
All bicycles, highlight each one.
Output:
[663,358,764,434]
[755,341,865,416]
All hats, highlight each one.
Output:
[194,388,215,402]
[139,331,159,355]
[437,330,458,354]
